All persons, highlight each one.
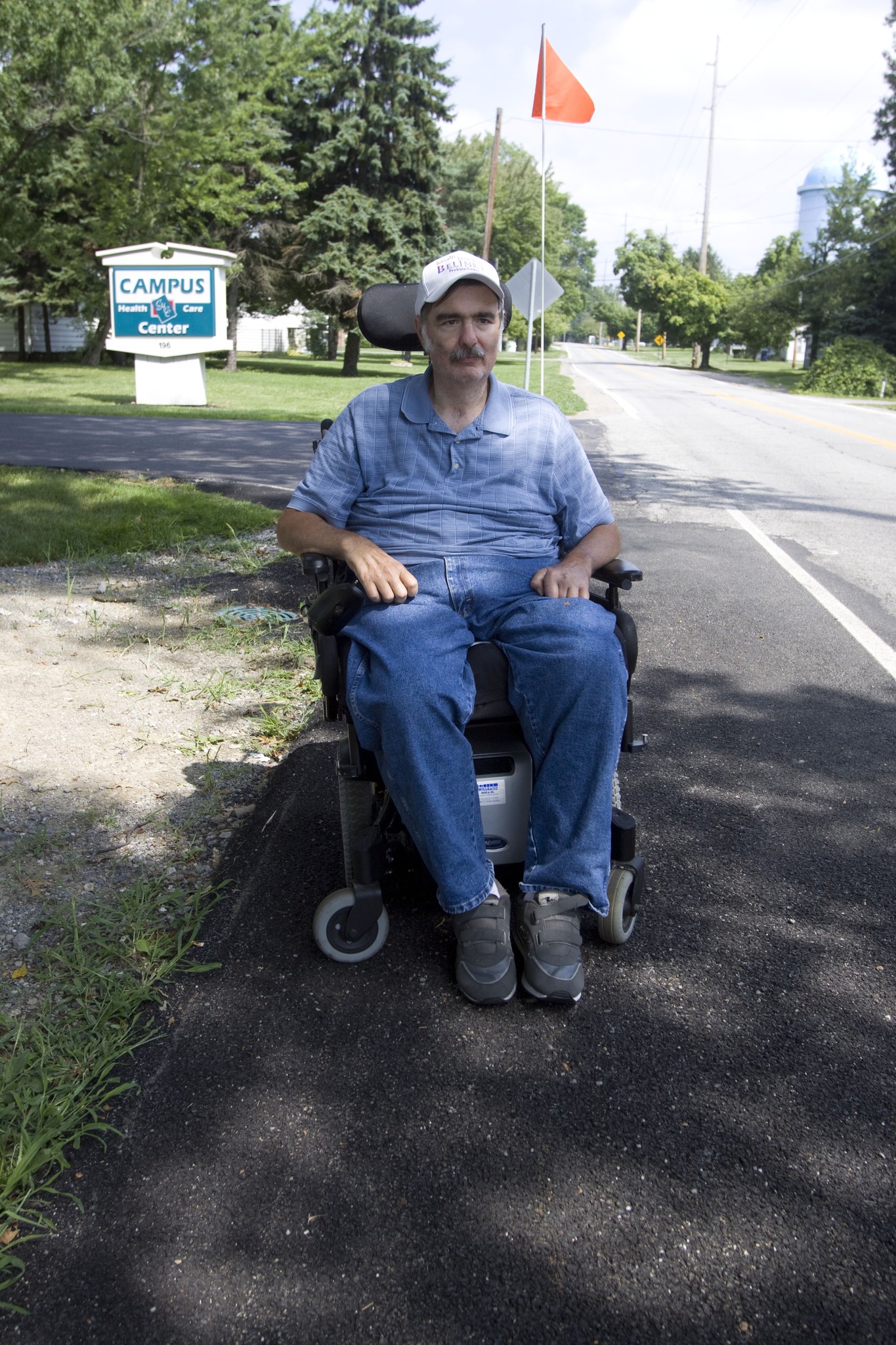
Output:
[278,250,626,1002]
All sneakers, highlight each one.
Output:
[513,888,588,1003]
[450,877,517,1003]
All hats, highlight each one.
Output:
[415,250,503,317]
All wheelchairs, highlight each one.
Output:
[299,284,644,960]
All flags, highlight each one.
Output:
[531,35,596,124]
